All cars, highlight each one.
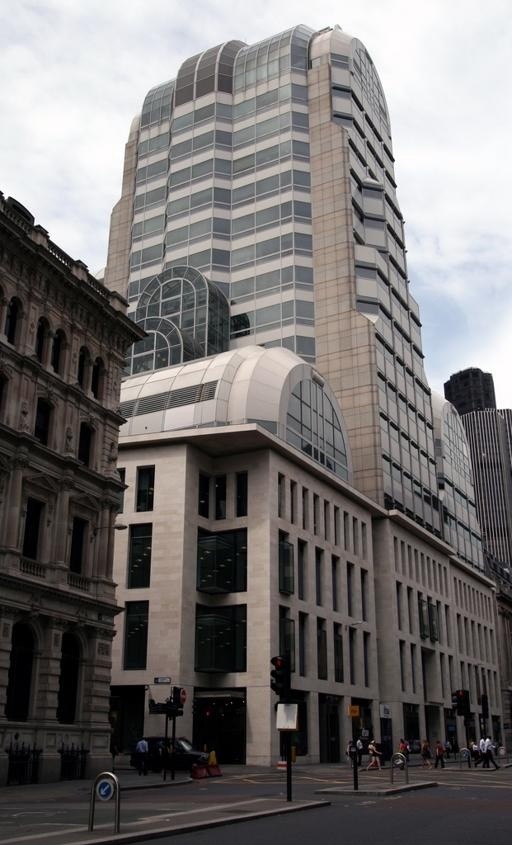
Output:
[130,736,211,773]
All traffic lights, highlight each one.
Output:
[174,686,186,719]
[455,689,469,717]
[270,653,288,700]
[450,691,458,717]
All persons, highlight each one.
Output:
[136,737,149,776]
[346,736,501,771]
[158,737,166,778]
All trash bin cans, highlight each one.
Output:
[57,742,90,781]
[5,741,43,786]
